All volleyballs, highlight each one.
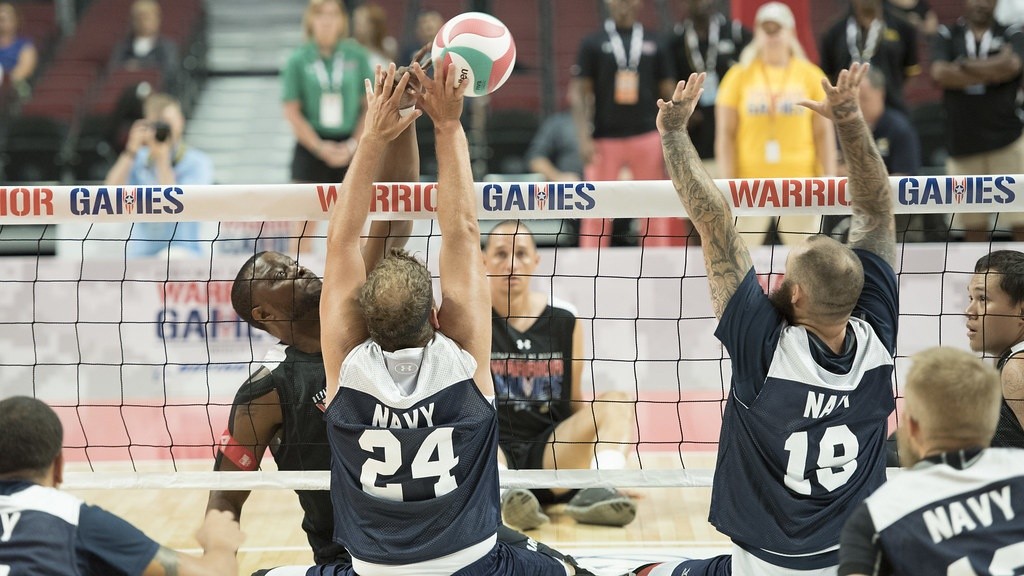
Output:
[431,12,517,97]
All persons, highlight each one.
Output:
[1,396,247,576]
[620,61,895,575]
[320,55,598,576]
[473,220,647,531]
[201,59,425,555]
[0,2,38,88]
[279,0,753,246]
[716,1,834,240]
[101,93,215,185]
[108,0,182,96]
[819,0,1024,242]
[962,249,1024,448]
[837,346,1024,575]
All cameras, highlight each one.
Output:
[149,122,170,143]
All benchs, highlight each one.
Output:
[0,0,211,186]
[356,0,997,163]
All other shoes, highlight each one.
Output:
[566,485,637,527]
[504,490,549,532]
